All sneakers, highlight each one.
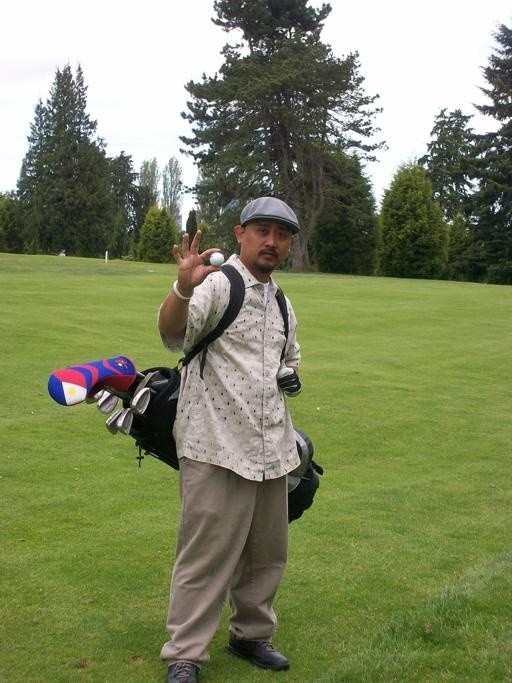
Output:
[166,662,201,683]
[228,639,290,670]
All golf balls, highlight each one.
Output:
[210,252,225,265]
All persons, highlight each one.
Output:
[156,195,304,682]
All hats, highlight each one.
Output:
[240,197,301,235]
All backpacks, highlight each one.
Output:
[123,264,323,524]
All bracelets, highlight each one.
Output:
[171,280,193,302]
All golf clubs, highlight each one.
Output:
[91,371,169,435]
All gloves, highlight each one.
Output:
[276,359,302,397]
